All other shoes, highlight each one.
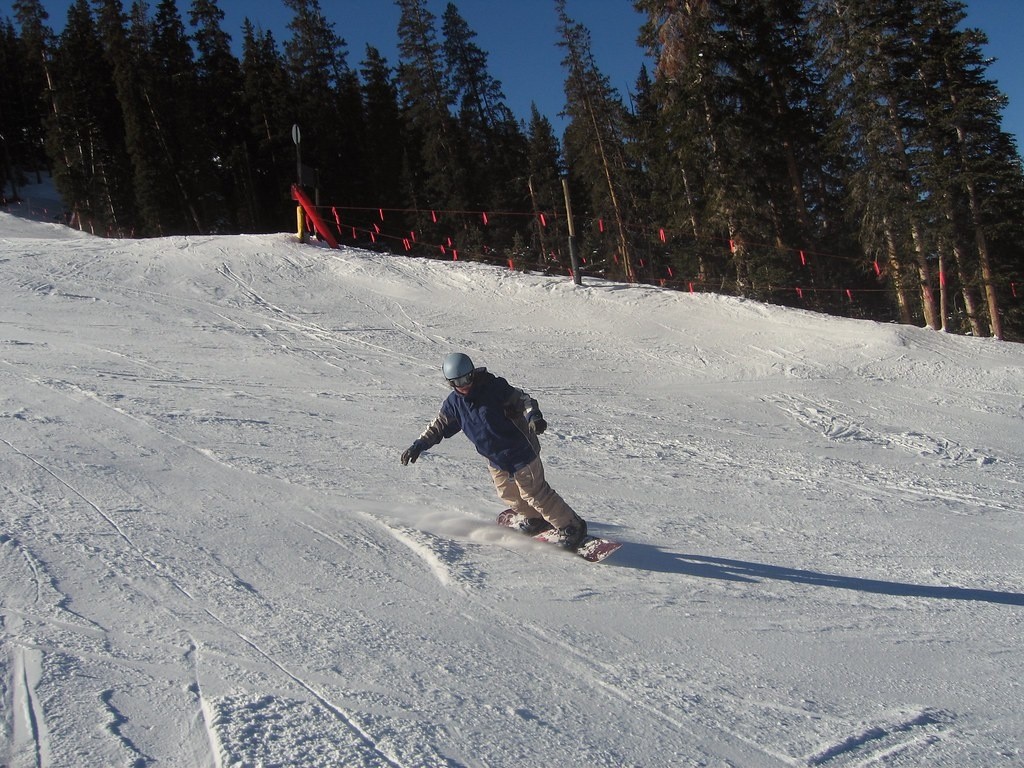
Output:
[518,516,546,537]
[558,516,587,550]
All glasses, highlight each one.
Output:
[446,369,474,387]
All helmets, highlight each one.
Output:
[441,353,474,380]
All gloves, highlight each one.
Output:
[526,410,547,435]
[401,439,430,466]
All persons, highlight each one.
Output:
[401,353,588,551]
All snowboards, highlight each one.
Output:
[494,508,624,564]
